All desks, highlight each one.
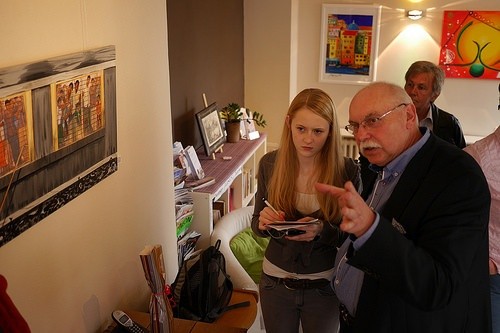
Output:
[102,288,260,333]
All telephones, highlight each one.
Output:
[112,309,144,333]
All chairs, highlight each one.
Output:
[210,205,270,333]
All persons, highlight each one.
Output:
[358,61,467,190]
[461,83,500,333]
[56,74,103,144]
[314,81,492,333]
[0,100,23,168]
[251,88,360,333]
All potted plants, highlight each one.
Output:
[221,102,268,143]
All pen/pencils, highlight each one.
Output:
[262,197,285,221]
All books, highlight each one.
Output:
[173,140,206,269]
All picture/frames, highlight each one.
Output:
[317,4,381,86]
[196,102,227,157]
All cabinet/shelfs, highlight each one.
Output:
[193,132,267,255]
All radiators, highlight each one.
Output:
[340,127,360,163]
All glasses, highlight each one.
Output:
[345,103,407,135]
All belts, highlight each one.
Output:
[339,304,355,328]
[262,269,330,290]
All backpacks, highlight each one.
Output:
[180,239,250,324]
